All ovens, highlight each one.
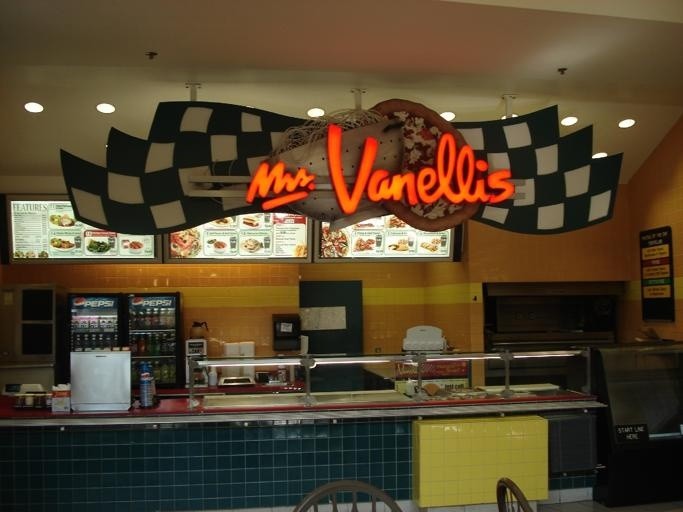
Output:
[481,283,623,471]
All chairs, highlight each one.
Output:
[494,475,535,512]
[292,480,404,511]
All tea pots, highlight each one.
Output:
[190,320,209,338]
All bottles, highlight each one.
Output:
[277,365,286,382]
[264,237,270,250]
[209,366,217,386]
[13,391,52,406]
[73,235,81,249]
[74,333,120,351]
[106,235,114,248]
[126,308,181,385]
[407,236,414,249]
[229,236,237,253]
[439,235,446,248]
[263,212,270,223]
[137,359,153,408]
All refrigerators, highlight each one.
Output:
[63,292,184,389]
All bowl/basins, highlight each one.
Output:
[128,242,144,254]
[213,241,226,252]
[206,238,214,247]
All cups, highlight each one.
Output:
[376,235,382,247]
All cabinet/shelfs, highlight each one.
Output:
[184,347,595,407]
[584,336,683,507]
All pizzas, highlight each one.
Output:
[358,98,483,232]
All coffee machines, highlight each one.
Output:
[186,338,207,391]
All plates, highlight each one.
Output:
[221,342,255,379]
[48,220,74,229]
[209,218,233,227]
[49,245,75,252]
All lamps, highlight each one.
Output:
[511,350,582,359]
[424,352,501,361]
[195,358,303,368]
[313,357,412,364]
